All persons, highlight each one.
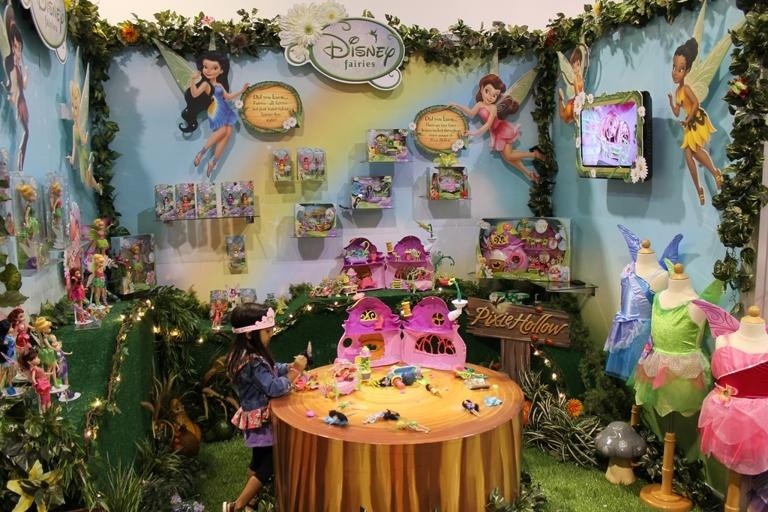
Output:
[1,3,31,171]
[222,301,309,512]
[625,257,715,416]
[1,182,158,418]
[665,1,750,206]
[445,47,547,186]
[555,43,591,124]
[149,30,251,178]
[63,45,104,196]
[596,112,619,165]
[690,296,768,476]
[604,223,685,382]
[615,121,632,147]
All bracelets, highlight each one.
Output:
[289,367,300,375]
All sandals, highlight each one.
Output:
[221,500,255,512]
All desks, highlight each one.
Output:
[269,361,525,511]
